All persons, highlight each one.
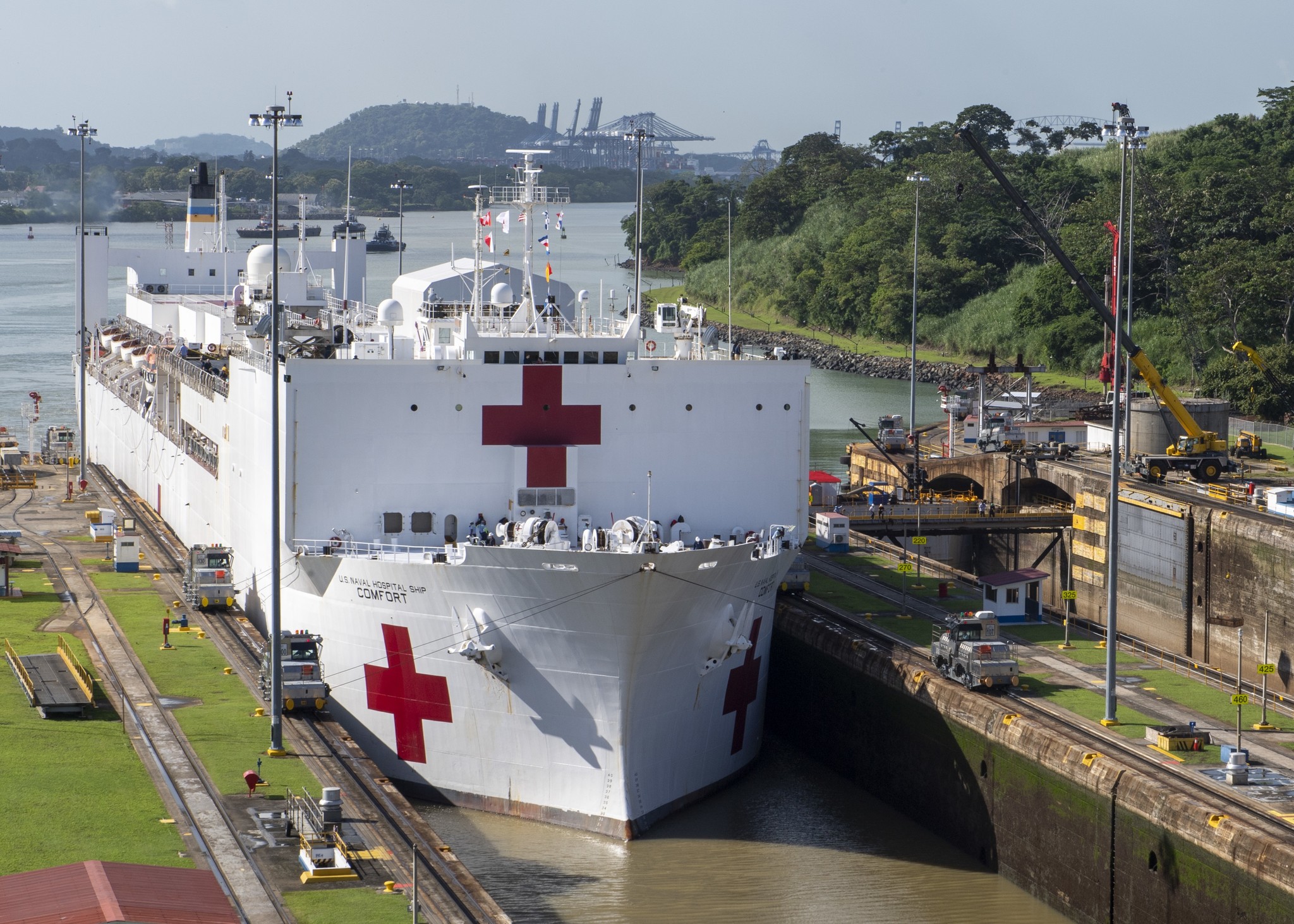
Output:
[731,342,742,360]
[558,518,567,531]
[693,536,704,550]
[278,354,286,364]
[353,355,358,359]
[649,529,657,540]
[469,520,496,547]
[199,358,228,380]
[294,650,311,656]
[772,527,781,552]
[583,522,590,531]
[979,502,996,517]
[297,345,304,358]
[179,343,188,360]
[218,559,224,567]
[963,631,968,639]
[792,349,801,360]
[524,354,544,364]
[324,340,332,359]
[869,503,885,519]
[833,504,843,514]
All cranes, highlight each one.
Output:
[517,97,717,171]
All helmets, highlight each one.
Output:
[880,504,883,506]
[695,537,700,542]
[872,504,875,506]
[488,532,493,536]
[835,506,838,509]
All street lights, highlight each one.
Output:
[66,121,97,495]
[906,171,931,448]
[248,107,306,760]
[624,127,656,314]
[1100,114,1149,728]
[390,179,413,276]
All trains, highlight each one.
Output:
[953,387,979,421]
[182,544,236,611]
[977,412,1026,453]
[257,629,332,715]
[878,415,906,454]
[931,611,1019,692]
[41,425,80,465]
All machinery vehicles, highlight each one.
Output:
[954,120,1239,485]
[1232,340,1294,427]
[1229,429,1267,460]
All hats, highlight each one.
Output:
[470,520,474,523]
[777,527,781,530]
[481,520,485,523]
[585,522,590,524]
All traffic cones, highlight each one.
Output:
[1193,737,1199,751]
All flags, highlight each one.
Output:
[480,210,493,255]
[496,208,564,233]
[544,288,552,316]
[538,235,552,283]
[502,248,510,276]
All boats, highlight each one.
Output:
[365,223,406,254]
[236,214,321,238]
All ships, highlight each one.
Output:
[70,148,811,841]
